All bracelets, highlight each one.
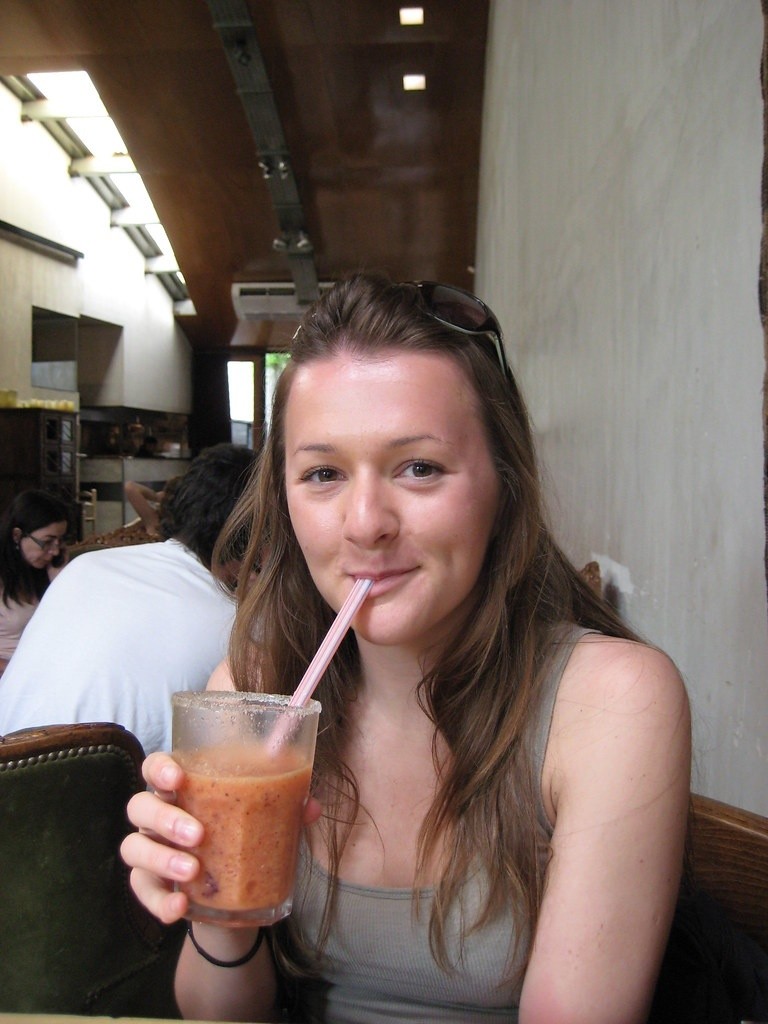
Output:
[187,922,264,967]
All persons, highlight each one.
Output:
[123,476,184,536]
[117,275,693,1024]
[0,443,259,784]
[0,489,65,674]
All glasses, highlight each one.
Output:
[24,531,66,551]
[293,279,507,380]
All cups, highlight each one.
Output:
[171,692,322,927]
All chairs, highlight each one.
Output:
[0,724,186,1021]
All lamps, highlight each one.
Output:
[294,229,314,255]
[272,226,291,251]
